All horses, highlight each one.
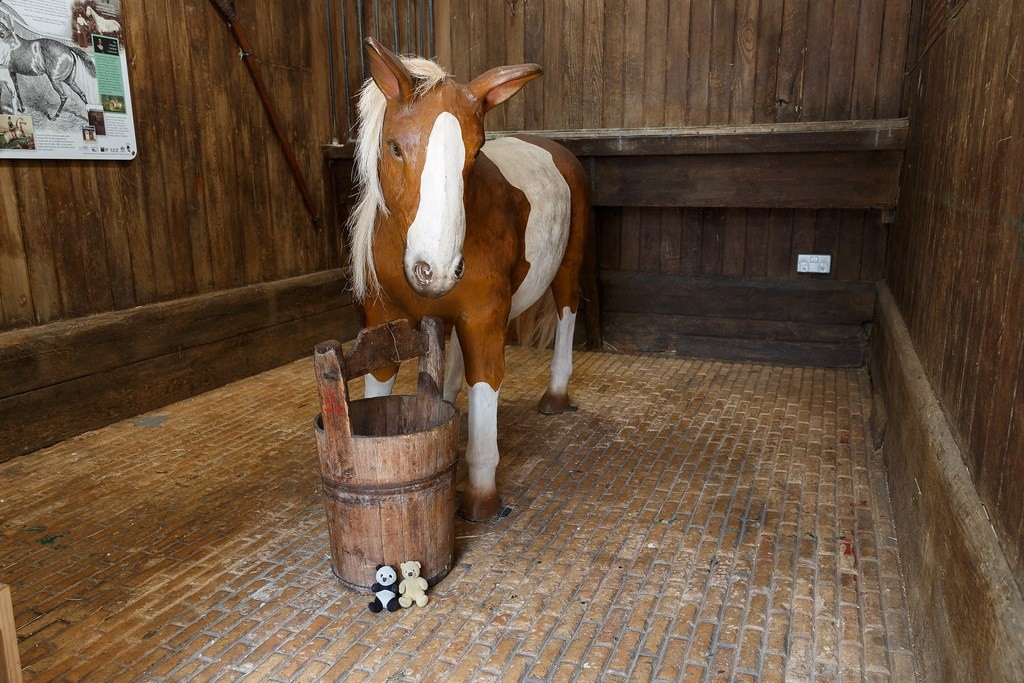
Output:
[341,35,589,523]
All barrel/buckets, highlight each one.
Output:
[314,316,457,595]
